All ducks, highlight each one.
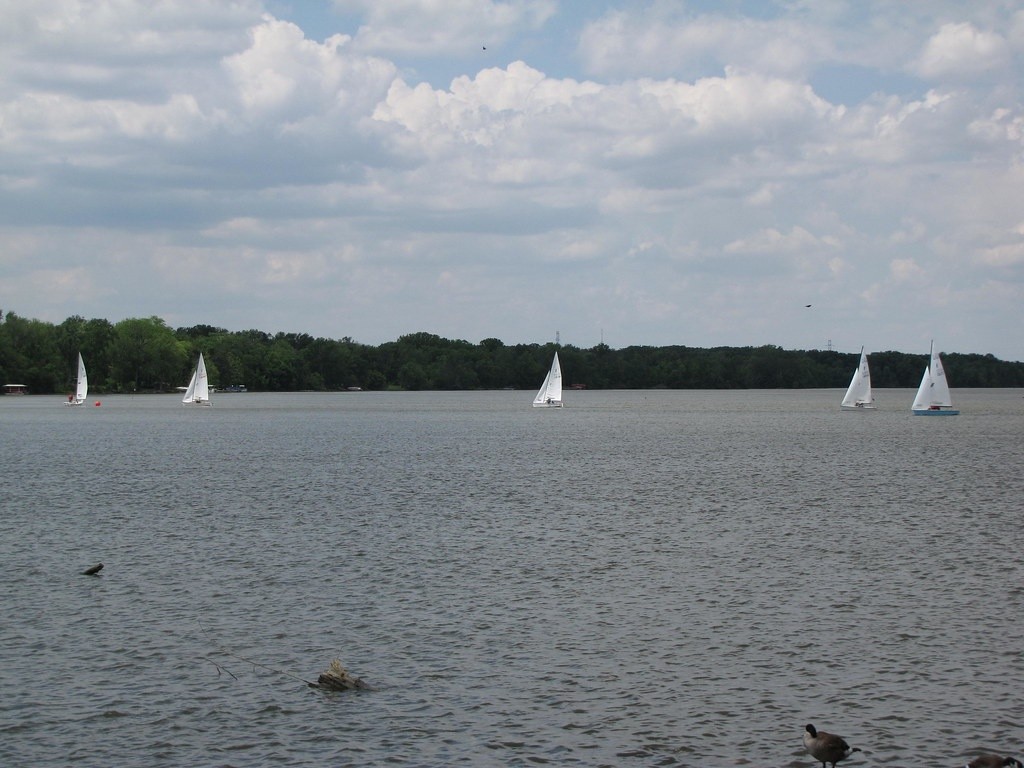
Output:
[959,752,1024,768]
[803,721,862,768]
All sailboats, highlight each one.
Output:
[910,339,960,415]
[63,352,88,406]
[532,351,564,408]
[181,352,213,407]
[840,346,877,411]
